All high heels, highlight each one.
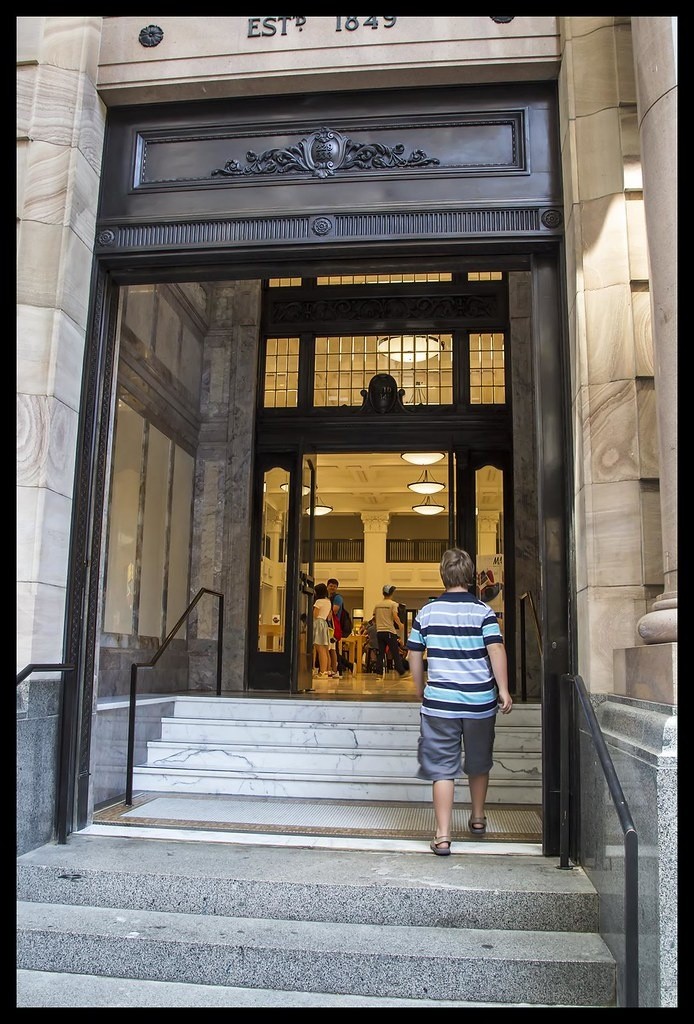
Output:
[315,671,328,679]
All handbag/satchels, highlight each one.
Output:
[331,594,352,638]
[329,627,334,638]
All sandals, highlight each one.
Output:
[469,815,486,834]
[430,836,452,854]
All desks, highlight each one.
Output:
[338,637,363,673]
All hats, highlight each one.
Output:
[383,585,396,594]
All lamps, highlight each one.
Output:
[401,382,445,465]
[412,495,444,515]
[305,495,333,516]
[378,335,444,362]
[407,469,446,495]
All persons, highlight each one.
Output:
[365,585,411,679]
[313,579,357,678]
[406,548,513,855]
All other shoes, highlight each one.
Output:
[332,671,340,678]
[376,678,383,682]
[339,676,343,680]
[400,673,412,680]
[351,662,357,678]
[328,671,332,678]
